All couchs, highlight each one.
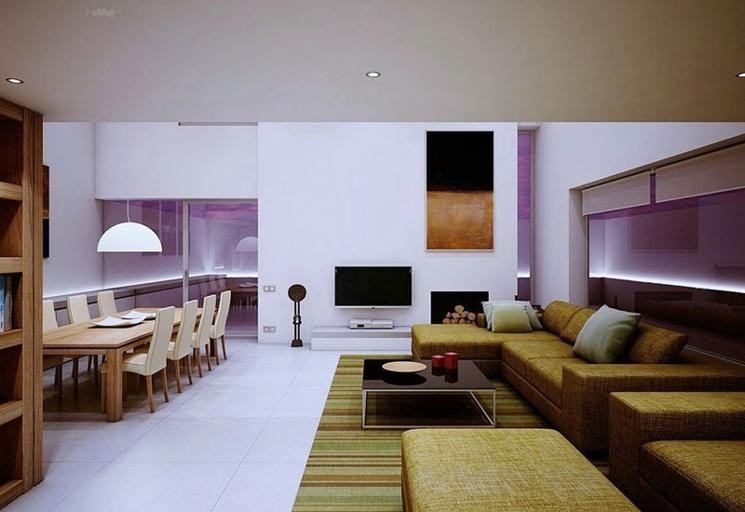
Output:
[412,300,745,451]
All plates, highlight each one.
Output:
[381,360,427,373]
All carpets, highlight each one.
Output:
[288,353,613,512]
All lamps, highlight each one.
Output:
[97,200,163,253]
[236,209,258,252]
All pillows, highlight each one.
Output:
[572,303,642,365]
[488,302,532,332]
[479,300,543,330]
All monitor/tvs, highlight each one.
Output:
[333,266,414,309]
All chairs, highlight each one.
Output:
[197,275,259,313]
[42,287,232,424]
[608,390,745,512]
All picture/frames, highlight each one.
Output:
[628,197,700,253]
[425,130,496,254]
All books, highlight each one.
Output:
[0,275,15,332]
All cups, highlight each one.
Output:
[431,367,446,377]
[444,352,458,370]
[444,370,459,383]
[432,355,444,366]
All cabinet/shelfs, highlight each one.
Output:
[0,97,44,509]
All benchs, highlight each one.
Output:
[399,426,639,511]
